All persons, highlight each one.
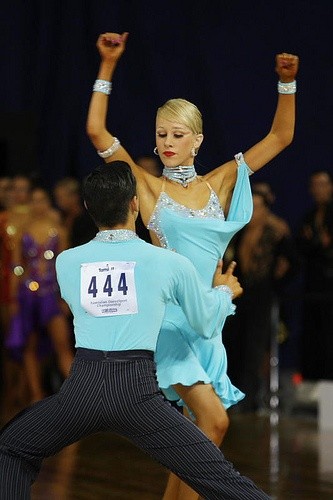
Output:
[85,32,299,500]
[0,160,272,500]
[0,174,98,406]
[223,169,332,413]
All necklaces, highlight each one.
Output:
[162,166,197,188]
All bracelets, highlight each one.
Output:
[92,79,112,95]
[277,80,296,95]
